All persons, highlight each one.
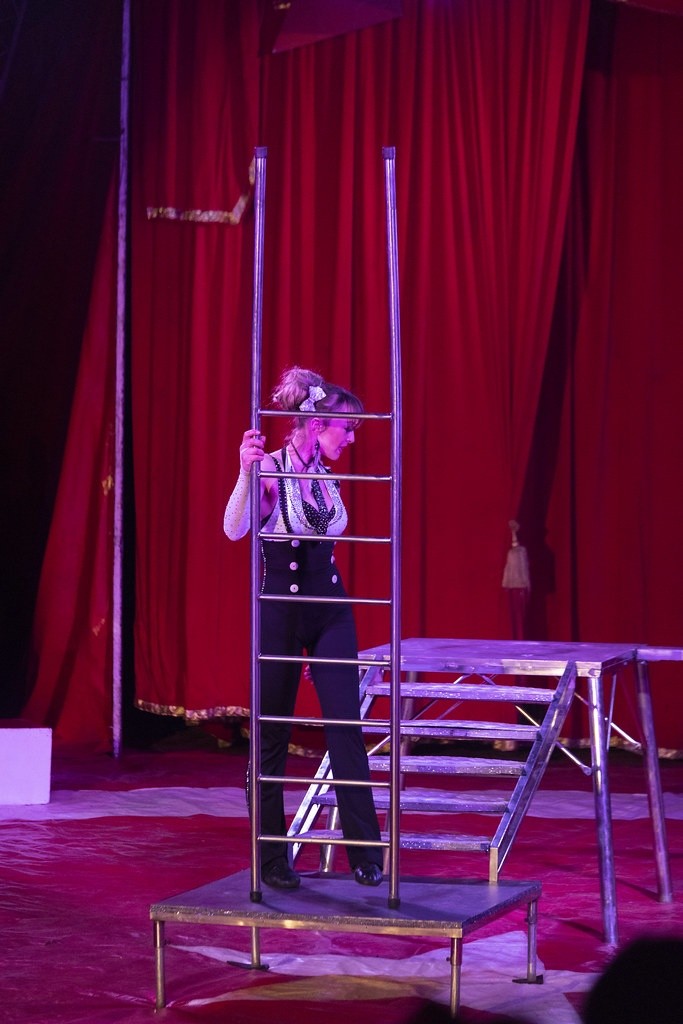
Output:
[223,367,386,890]
[583,935,683,1024]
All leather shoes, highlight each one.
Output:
[354,862,383,886]
[263,864,300,888]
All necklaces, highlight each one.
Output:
[290,440,316,472]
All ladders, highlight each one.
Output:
[247,144,404,910]
